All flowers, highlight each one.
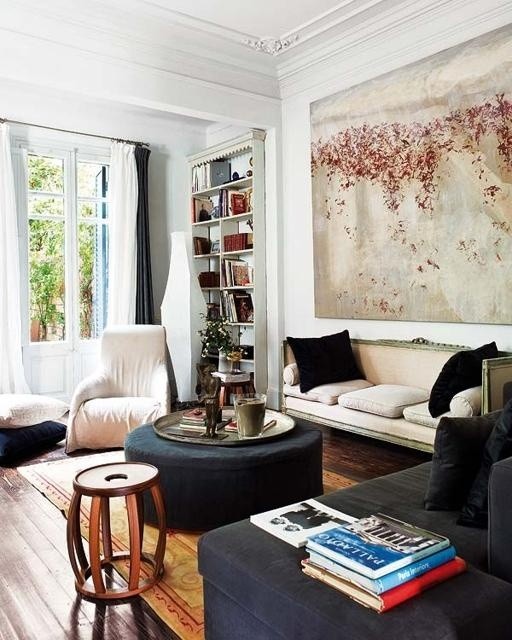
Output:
[191,310,247,359]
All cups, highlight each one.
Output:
[233,393,267,438]
[219,349,232,374]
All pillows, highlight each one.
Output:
[286,329,365,396]
[1,421,68,469]
[427,338,500,419]
[281,363,300,387]
[455,395,512,533]
[1,392,72,432]
[421,407,504,513]
[448,385,485,419]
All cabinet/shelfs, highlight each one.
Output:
[184,127,269,396]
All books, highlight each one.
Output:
[191,162,254,324]
[249,499,468,614]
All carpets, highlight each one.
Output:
[11,446,364,639]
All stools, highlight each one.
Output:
[208,370,257,407]
[64,460,170,604]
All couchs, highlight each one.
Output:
[193,452,511,638]
[275,332,512,460]
[60,323,174,455]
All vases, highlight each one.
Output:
[217,348,239,373]
